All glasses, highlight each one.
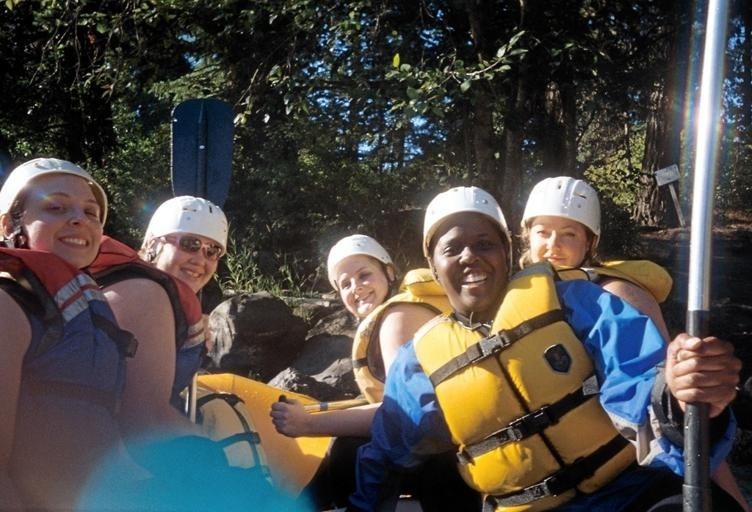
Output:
[165,235,224,261]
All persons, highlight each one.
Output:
[517,177,750,511]
[0,157,129,511]
[345,186,749,512]
[268,234,481,511]
[101,195,229,472]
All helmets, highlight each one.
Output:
[327,234,396,291]
[424,186,513,286]
[145,196,229,255]
[521,177,601,248]
[0,158,108,248]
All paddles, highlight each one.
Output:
[170,98,233,423]
